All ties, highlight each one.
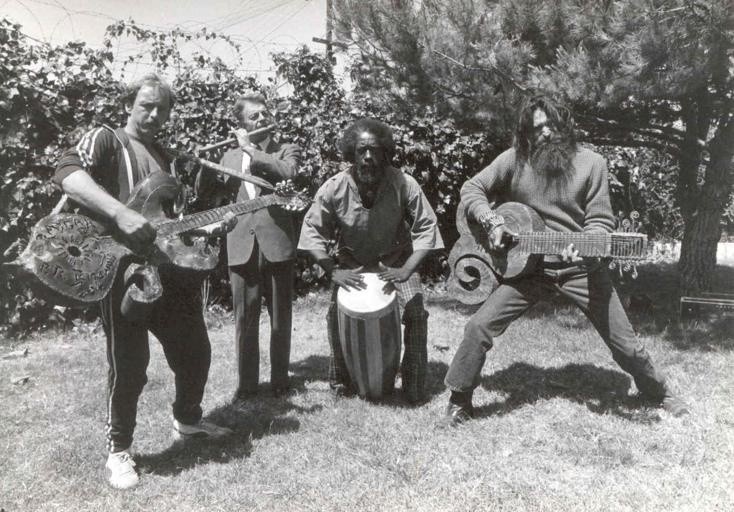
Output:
[250,145,263,199]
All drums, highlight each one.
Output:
[335,271,402,399]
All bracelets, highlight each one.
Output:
[481,215,504,235]
[479,210,495,223]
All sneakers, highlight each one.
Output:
[103,447,141,490]
[648,386,692,417]
[169,417,234,438]
[232,387,259,404]
[435,399,474,430]
[272,384,292,400]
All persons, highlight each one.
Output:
[442,93,693,421]
[292,116,445,405]
[193,92,301,397]
[56,77,230,491]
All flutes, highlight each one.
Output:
[197,125,273,155]
